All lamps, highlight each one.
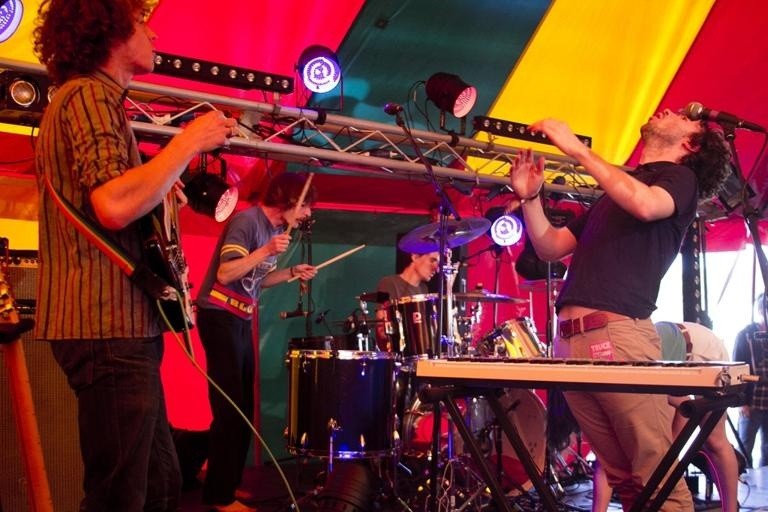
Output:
[292,45,345,113]
[422,72,477,134]
[487,207,525,247]
[182,152,241,223]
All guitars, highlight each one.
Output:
[141,185,196,333]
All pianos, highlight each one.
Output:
[415,352,751,398]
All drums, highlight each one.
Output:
[285,348,397,460]
[389,293,462,361]
[404,386,546,501]
[476,316,546,359]
[288,334,344,350]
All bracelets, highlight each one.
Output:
[518,193,540,204]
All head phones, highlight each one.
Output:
[342,308,369,336]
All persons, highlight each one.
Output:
[369,252,441,454]
[33,0,239,512]
[509,108,733,512]
[195,172,318,512]
[592,321,739,511]
[733,292,768,469]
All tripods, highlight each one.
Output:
[434,206,595,512]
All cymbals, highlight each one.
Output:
[399,217,492,254]
[519,279,565,293]
[453,290,530,303]
[332,317,383,327]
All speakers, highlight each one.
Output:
[0,70,63,127]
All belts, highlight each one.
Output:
[556,309,633,339]
[676,322,694,362]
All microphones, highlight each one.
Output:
[685,101,766,134]
[280,311,311,319]
[383,103,403,116]
[316,310,328,324]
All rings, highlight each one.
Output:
[230,127,234,136]
